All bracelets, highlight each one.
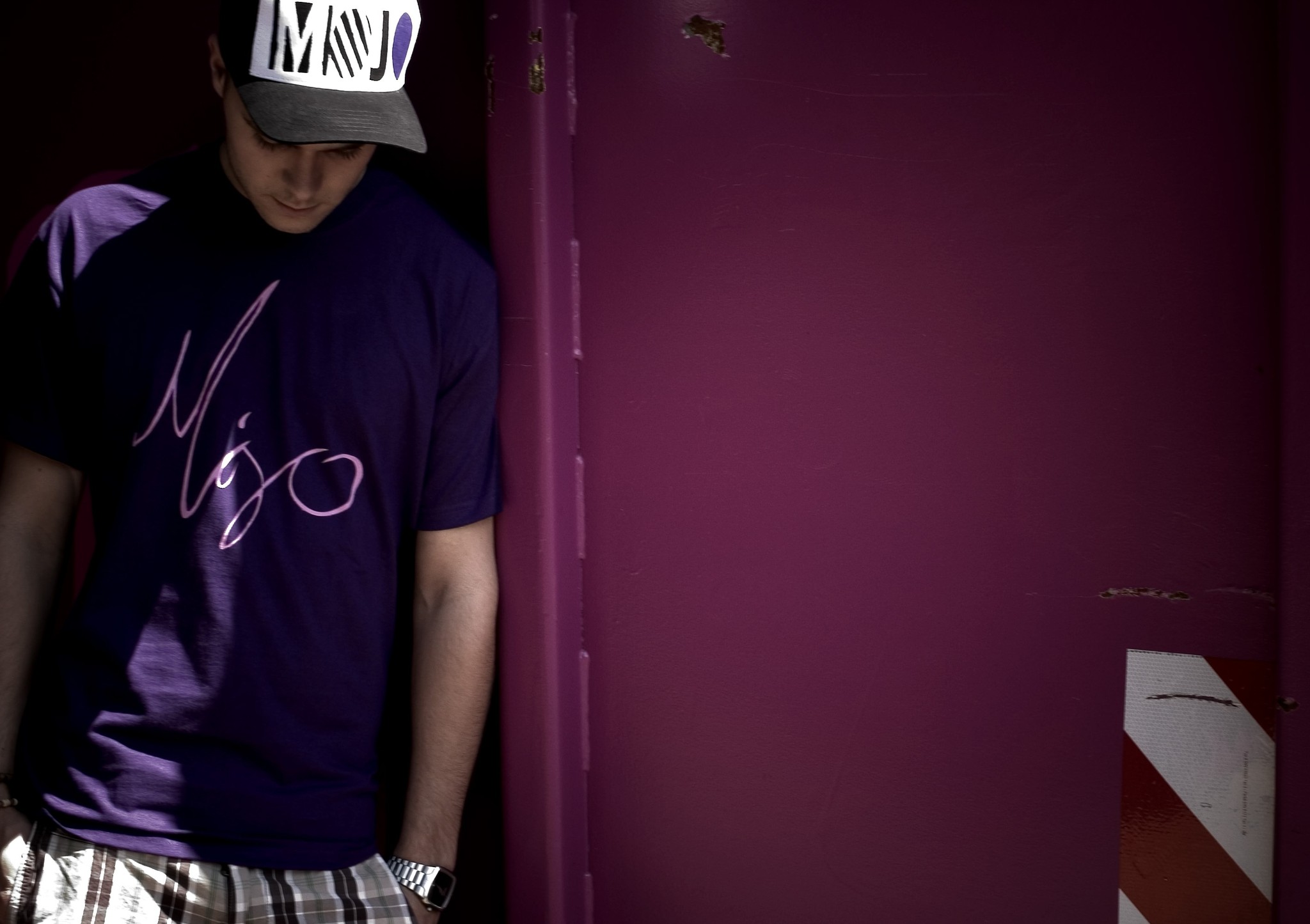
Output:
[1,799,23,807]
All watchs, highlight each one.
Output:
[388,855,457,912]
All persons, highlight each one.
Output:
[0,1,503,924]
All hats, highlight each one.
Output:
[206,0,430,157]
